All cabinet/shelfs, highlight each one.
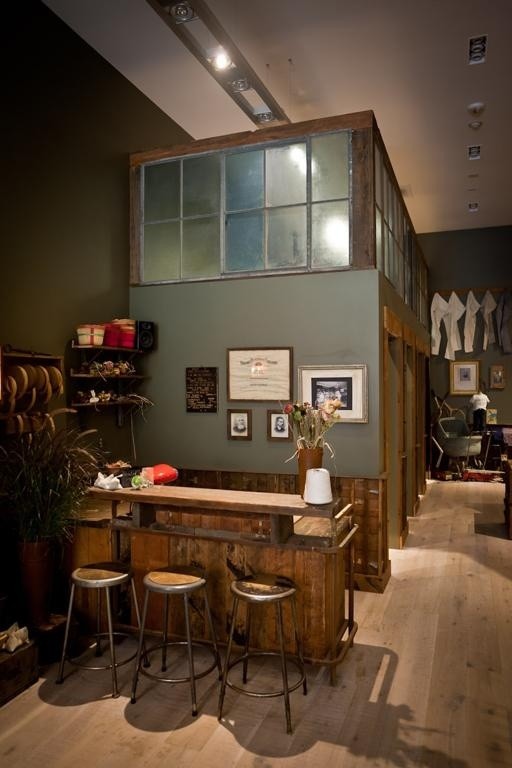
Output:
[67,340,150,409]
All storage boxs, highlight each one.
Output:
[0,641,38,708]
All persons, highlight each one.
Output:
[232,416,246,434]
[275,417,285,432]
[469,390,490,430]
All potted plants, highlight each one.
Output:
[0,429,110,628]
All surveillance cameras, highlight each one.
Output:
[466,103,485,117]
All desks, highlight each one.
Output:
[54,484,360,688]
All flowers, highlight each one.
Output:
[281,398,341,463]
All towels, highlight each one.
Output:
[429,292,456,361]
[480,289,498,351]
[463,290,483,353]
[495,293,512,354]
[448,290,466,352]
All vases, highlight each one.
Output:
[297,447,323,499]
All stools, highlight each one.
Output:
[217,575,308,737]
[55,562,150,699]
[131,566,224,718]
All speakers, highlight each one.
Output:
[135,320,156,350]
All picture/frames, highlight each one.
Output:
[227,408,252,441]
[449,361,479,396]
[226,346,293,403]
[297,364,369,424]
[267,409,293,442]
